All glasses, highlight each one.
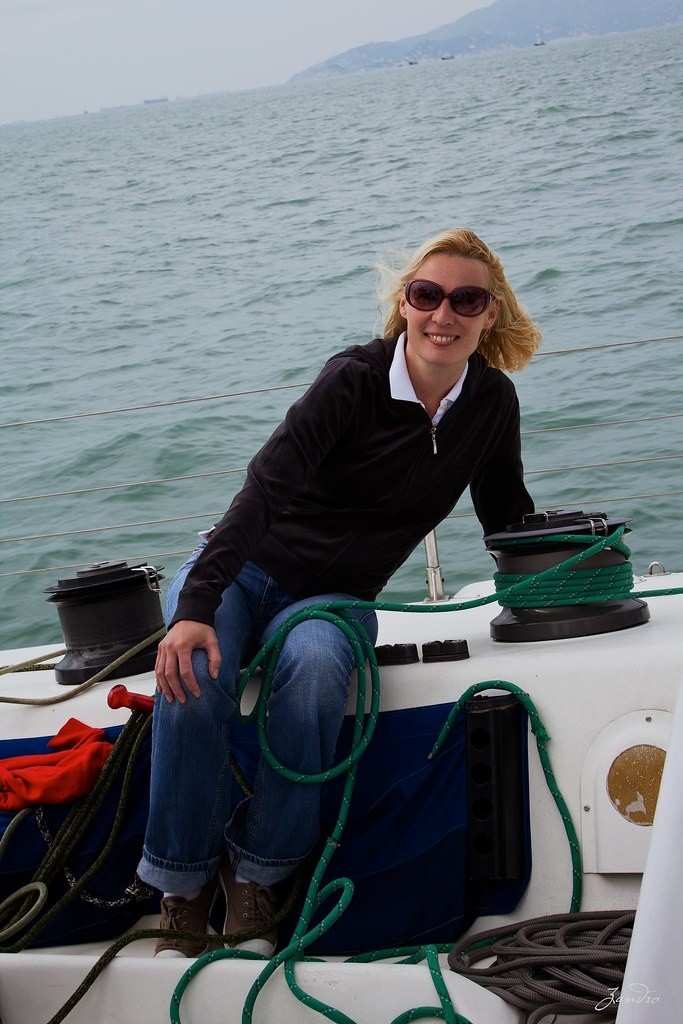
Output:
[406,279,496,318]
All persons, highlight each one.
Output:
[135,229,539,957]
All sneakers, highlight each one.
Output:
[154,864,219,959]
[219,859,278,957]
